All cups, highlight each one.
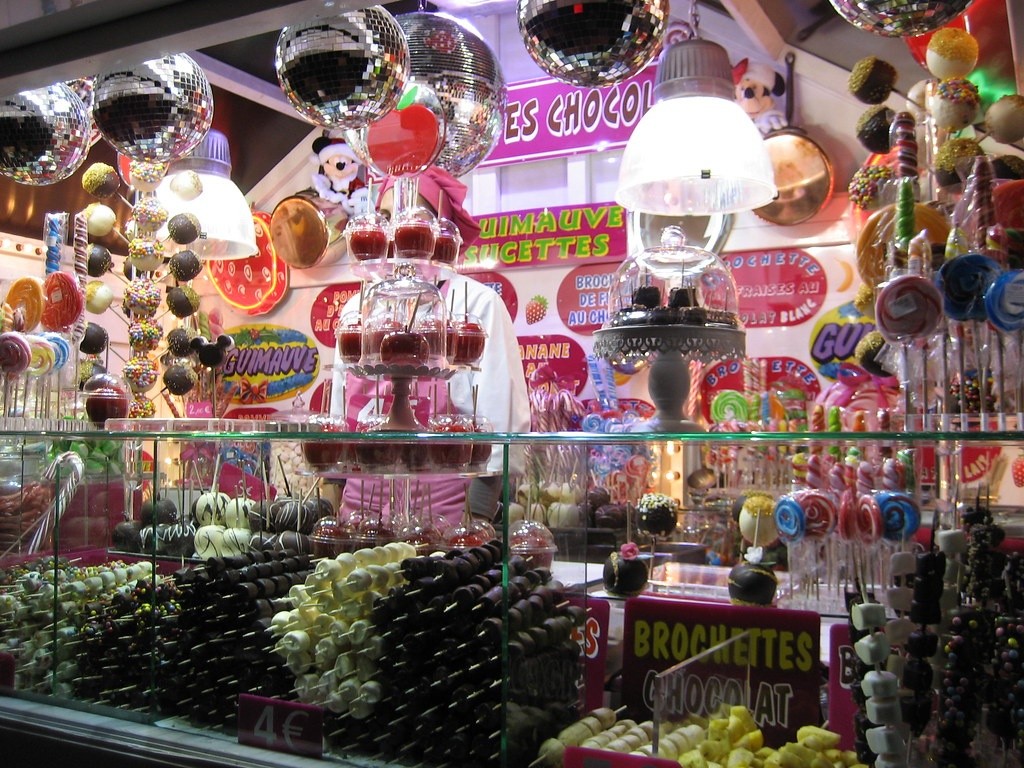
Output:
[342,207,464,267]
[307,507,557,566]
[334,309,486,366]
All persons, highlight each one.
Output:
[329,163,531,532]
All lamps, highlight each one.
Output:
[274,4,410,130]
[92,52,214,163]
[64,74,103,147]
[614,0,779,217]
[516,0,670,89]
[342,0,507,179]
[829,0,974,38]
[0,81,93,186]
[124,125,258,262]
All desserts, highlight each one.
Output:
[502,480,680,538]
[601,540,648,597]
[613,285,739,327]
[109,493,334,560]
[340,318,485,368]
[728,546,777,607]
[350,219,459,267]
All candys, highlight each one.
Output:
[0,161,236,418]
[708,27,1024,548]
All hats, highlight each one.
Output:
[374,165,482,257]
